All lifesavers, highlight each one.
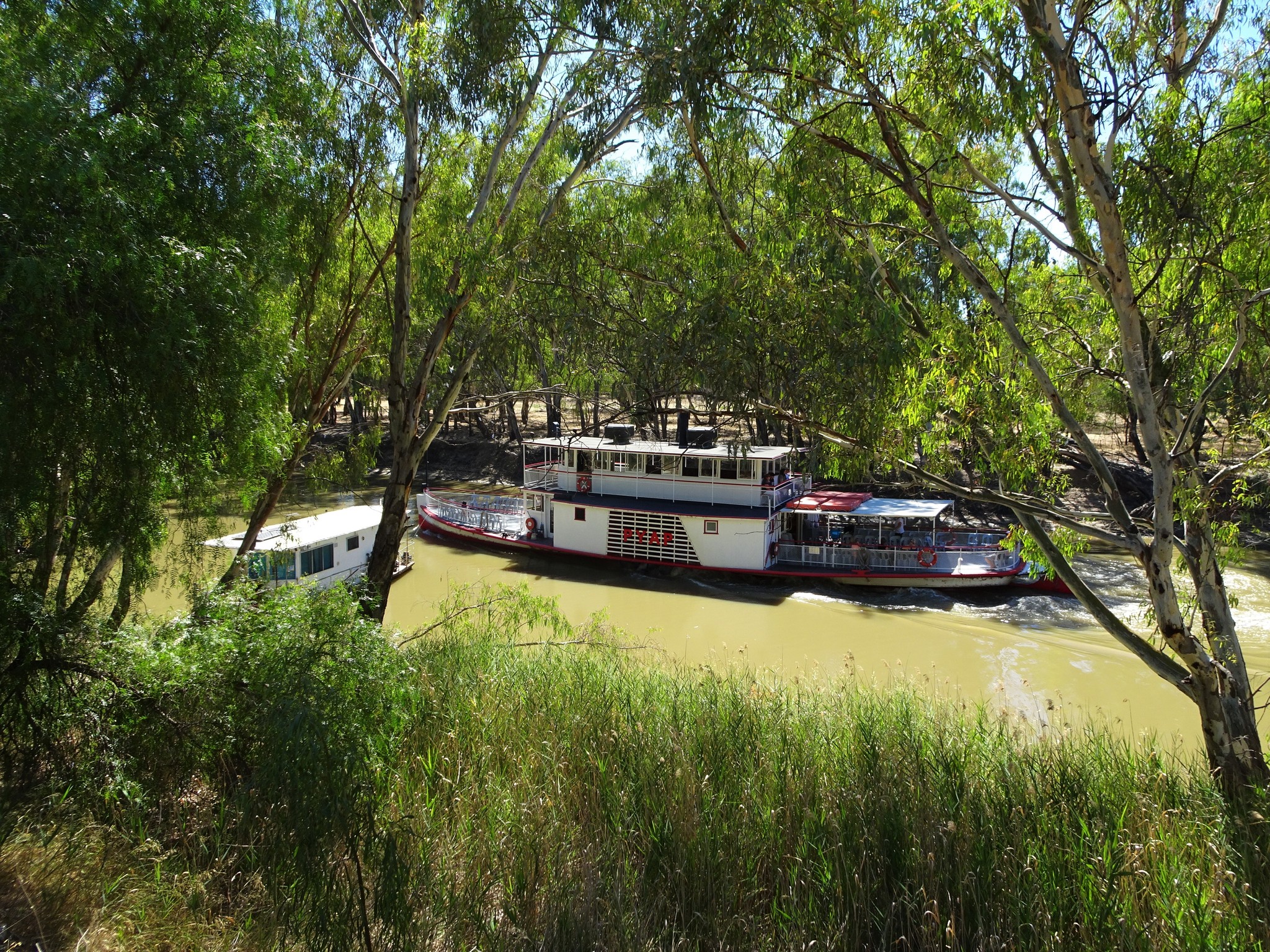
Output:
[577,476,591,493]
[917,547,937,567]
[940,528,957,546]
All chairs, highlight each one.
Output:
[980,533,993,549]
[993,550,1007,572]
[966,533,979,551]
[934,530,947,553]
[439,504,487,529]
[487,513,497,529]
[491,496,501,512]
[501,497,524,513]
[779,529,933,569]
[481,495,491,511]
[469,494,479,510]
[495,515,508,531]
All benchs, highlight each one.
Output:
[761,493,770,506]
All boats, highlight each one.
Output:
[417,425,1068,587]
[200,500,415,607]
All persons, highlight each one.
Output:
[647,455,661,466]
[877,537,890,559]
[804,516,813,526]
[577,450,585,472]
[769,473,777,490]
[841,530,851,544]
[778,469,795,498]
[851,540,861,558]
[902,538,919,559]
[701,459,712,476]
[785,467,800,496]
[818,514,826,526]
[761,474,782,506]
[995,531,1019,566]
[893,517,905,549]
[984,536,1005,571]
